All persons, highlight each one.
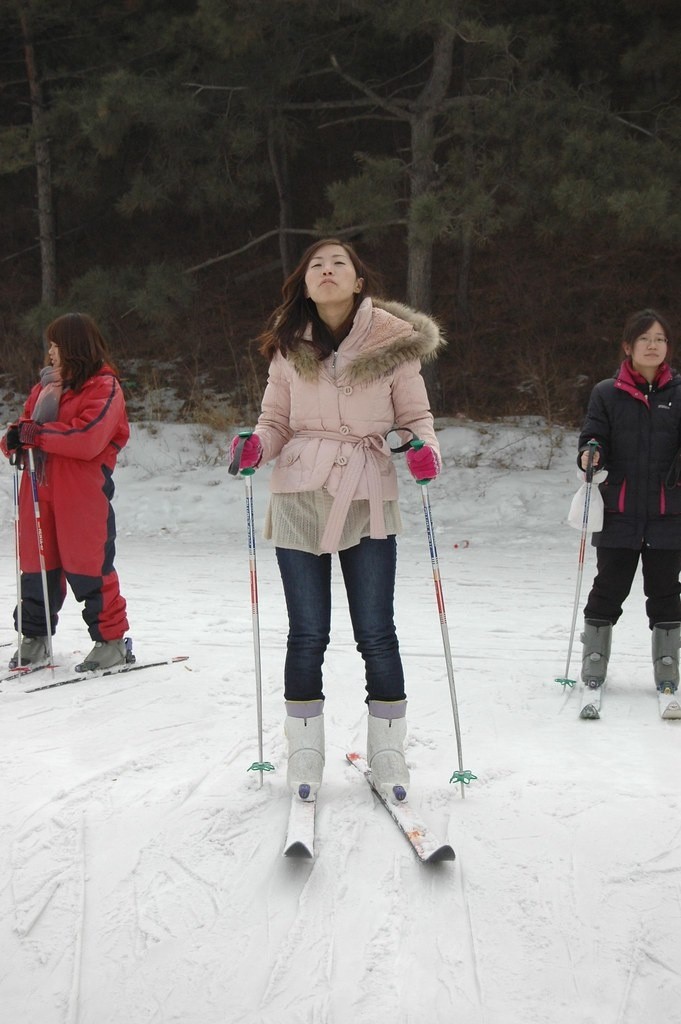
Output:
[229,239,446,803]
[0,312,136,675]
[576,309,681,694]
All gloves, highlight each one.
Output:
[17,421,37,444]
[228,432,263,469]
[405,445,440,481]
[5,428,20,451]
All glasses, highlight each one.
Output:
[635,335,669,344]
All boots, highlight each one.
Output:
[651,621,681,695]
[365,700,410,804]
[283,700,326,801]
[579,618,614,690]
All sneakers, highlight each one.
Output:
[74,637,136,673]
[8,635,54,672]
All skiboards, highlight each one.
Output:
[0,642,12,648]
[579,684,681,720]
[282,749,458,864]
[0,648,190,695]
